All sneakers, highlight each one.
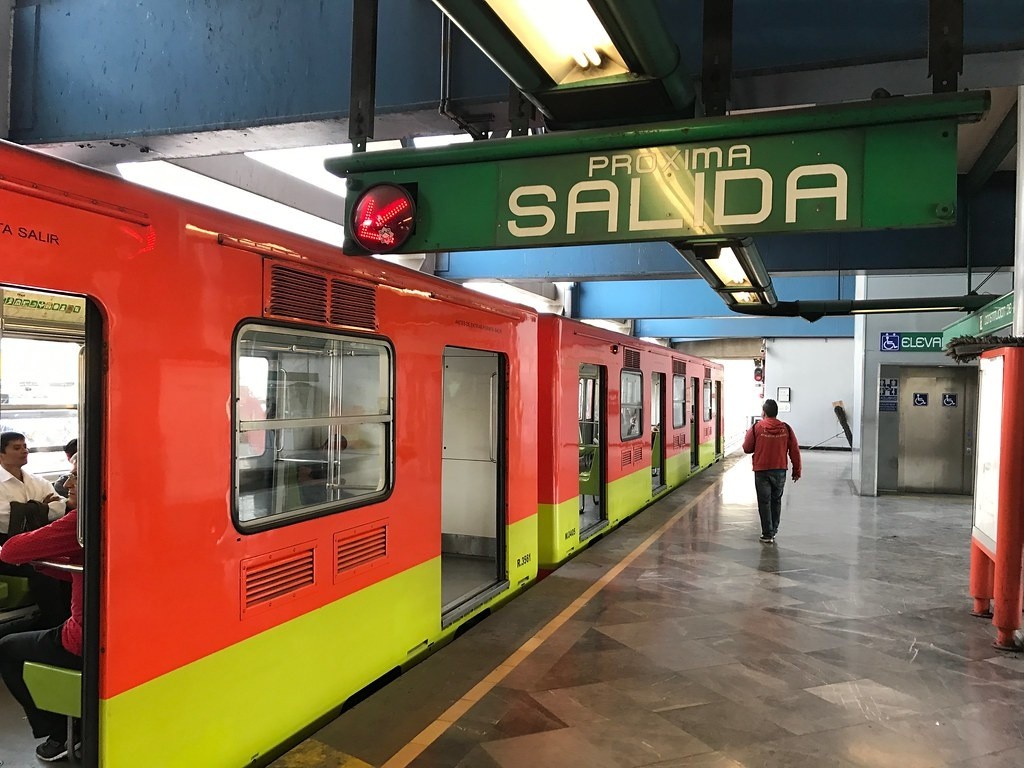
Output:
[35,735,81,762]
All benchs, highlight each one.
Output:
[23,661,81,764]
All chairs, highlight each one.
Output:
[652,431,661,477]
[578,427,593,458]
[579,445,599,514]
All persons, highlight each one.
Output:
[0,432,85,761]
[298,434,352,504]
[742,399,802,544]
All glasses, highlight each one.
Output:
[68,471,77,480]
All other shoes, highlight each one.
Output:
[772,532,776,539]
[759,535,773,542]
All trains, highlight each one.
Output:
[1,140,729,768]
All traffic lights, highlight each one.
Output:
[754,368,762,382]
[351,180,416,252]
